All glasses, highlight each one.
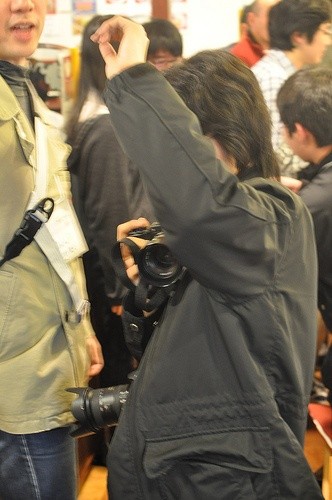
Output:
[150,59,177,67]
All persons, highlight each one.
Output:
[0,0,332,500]
[90,15,326,500]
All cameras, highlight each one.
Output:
[128,220,184,287]
[65,371,138,439]
